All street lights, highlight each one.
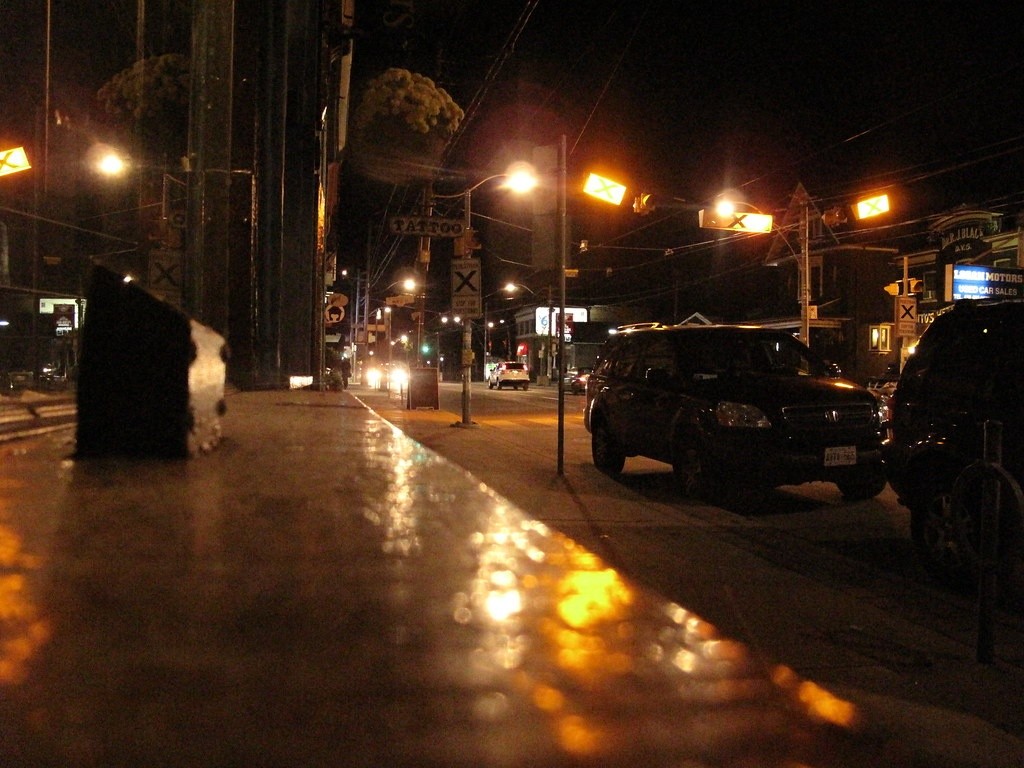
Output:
[715,191,891,349]
[432,162,538,429]
[96,150,282,386]
[506,135,627,479]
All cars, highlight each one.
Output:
[557,367,593,394]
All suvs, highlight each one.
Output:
[488,362,531,392]
[585,324,889,518]
[880,301,1024,579]
